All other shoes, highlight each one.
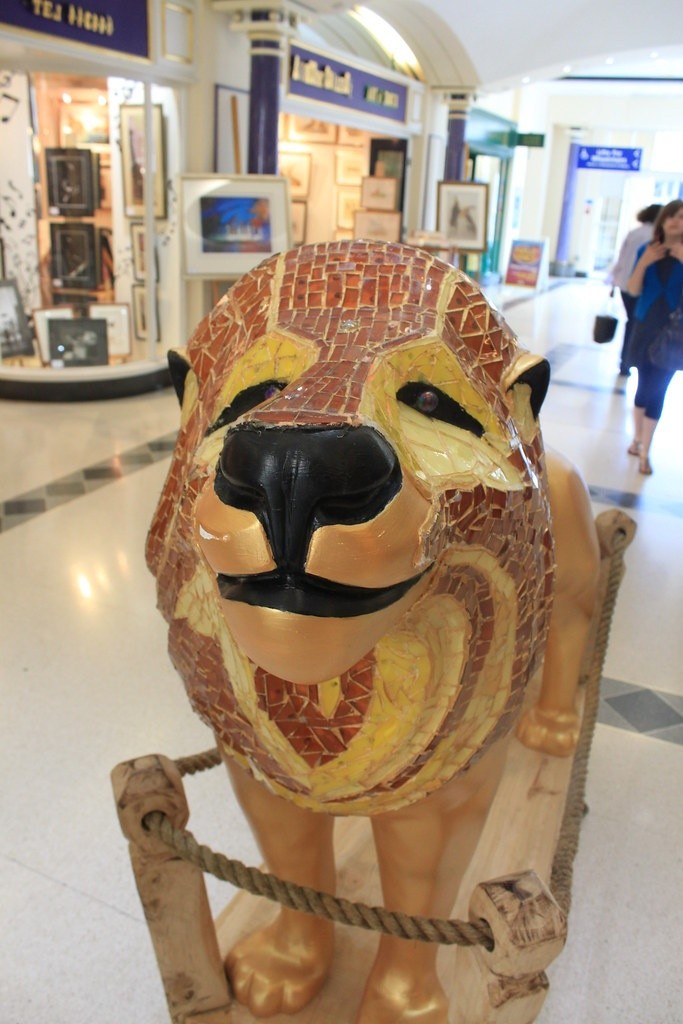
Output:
[614,371,628,393]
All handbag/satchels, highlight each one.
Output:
[647,310,682,371]
[592,315,618,343]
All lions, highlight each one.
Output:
[144,240,604,1024]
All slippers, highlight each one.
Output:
[629,437,640,455]
[640,456,651,474]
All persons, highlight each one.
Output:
[610,199,683,475]
